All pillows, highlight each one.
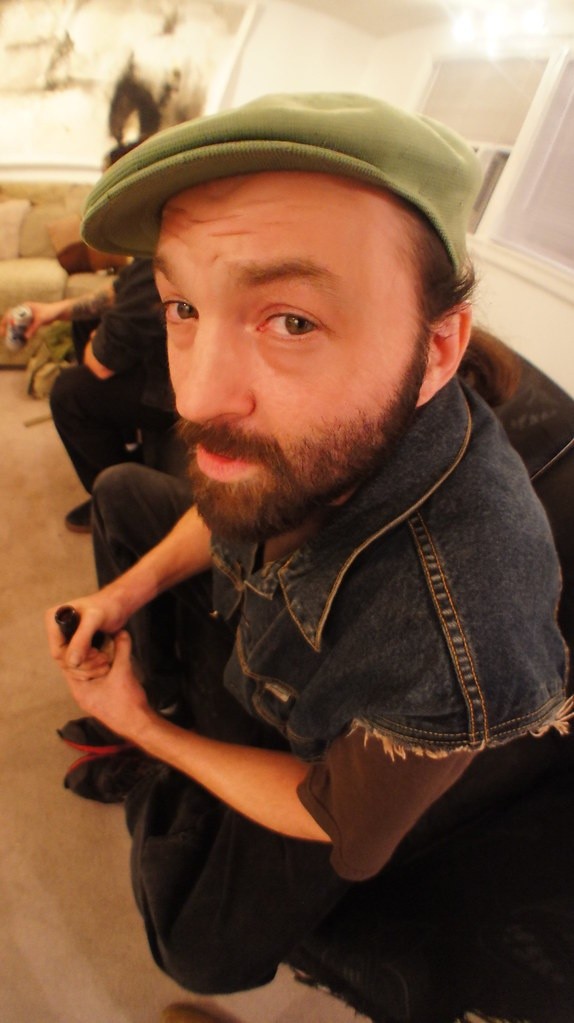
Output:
[1,197,84,260]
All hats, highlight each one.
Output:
[79,92,482,279]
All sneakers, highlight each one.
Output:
[56,718,137,753]
[63,754,137,803]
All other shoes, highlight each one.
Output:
[64,499,92,533]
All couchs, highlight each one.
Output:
[0,183,117,366]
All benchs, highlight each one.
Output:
[278,325,573,1023]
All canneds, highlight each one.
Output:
[6,307,32,351]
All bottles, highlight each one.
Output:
[55,605,169,711]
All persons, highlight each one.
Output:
[44,93,574,995]
[0,144,184,532]
